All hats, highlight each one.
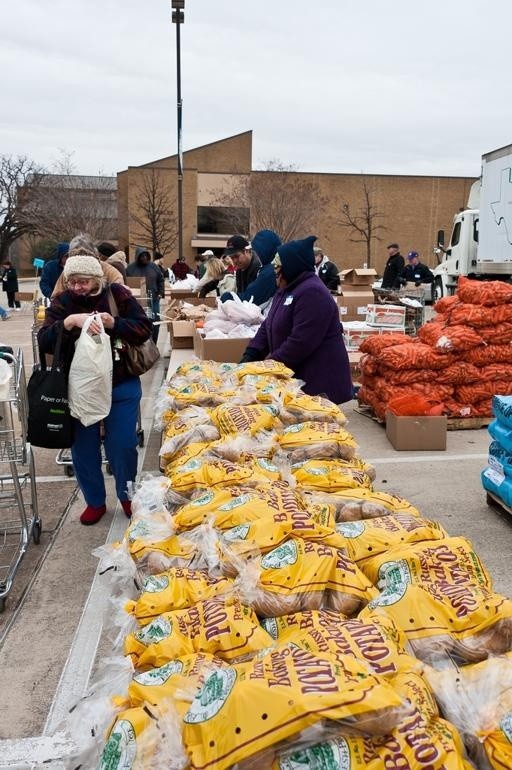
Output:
[313,246,323,254]
[64,256,104,281]
[408,251,419,259]
[225,236,249,255]
[58,243,69,257]
[388,244,399,249]
[270,252,282,266]
[201,250,214,256]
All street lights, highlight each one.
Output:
[171,0,190,270]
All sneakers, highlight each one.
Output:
[121,500,132,518]
[2,306,21,320]
[80,505,105,525]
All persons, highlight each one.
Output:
[195,231,282,305]
[0,306,11,320]
[171,256,191,280]
[396,251,434,330]
[0,261,21,311]
[40,235,169,344]
[240,235,354,405]
[381,243,404,290]
[314,247,339,292]
[37,247,155,525]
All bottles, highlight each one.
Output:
[36,305,47,320]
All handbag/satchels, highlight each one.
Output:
[26,370,76,448]
[117,334,161,375]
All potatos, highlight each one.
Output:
[131,358,511,770]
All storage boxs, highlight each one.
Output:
[152,288,253,364]
[126,277,147,312]
[14,292,34,301]
[337,268,407,350]
[384,411,447,451]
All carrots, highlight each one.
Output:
[356,276,511,418]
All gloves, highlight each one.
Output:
[415,279,421,286]
[400,277,406,285]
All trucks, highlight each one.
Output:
[426,145,512,308]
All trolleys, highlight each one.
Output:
[0,341,43,618]
[26,297,146,479]
